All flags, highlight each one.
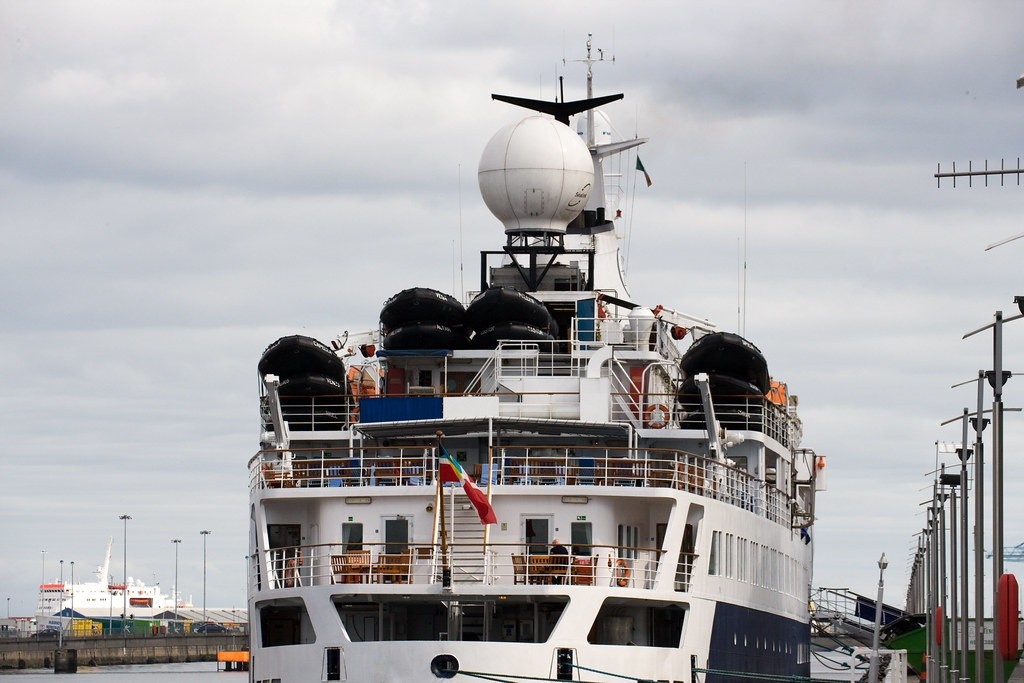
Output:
[438,441,499,526]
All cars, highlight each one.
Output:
[30,629,60,638]
[194,623,230,633]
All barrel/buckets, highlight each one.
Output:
[54,649,77,673]
[70,619,102,636]
[600,322,632,343]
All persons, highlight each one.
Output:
[537,539,571,585]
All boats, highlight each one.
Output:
[245,33,829,683]
[37,537,194,619]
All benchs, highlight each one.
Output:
[261,458,431,487]
[511,552,599,585]
[507,457,722,499]
[330,549,415,584]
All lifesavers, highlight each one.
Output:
[644,403,671,428]
[614,556,631,588]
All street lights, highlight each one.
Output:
[867,552,888,683]
[200,530,211,632]
[40,550,49,614]
[906,311,1024,683]
[119,515,132,631]
[171,539,181,619]
[71,561,74,636]
[59,560,64,647]
[8,598,11,619]
